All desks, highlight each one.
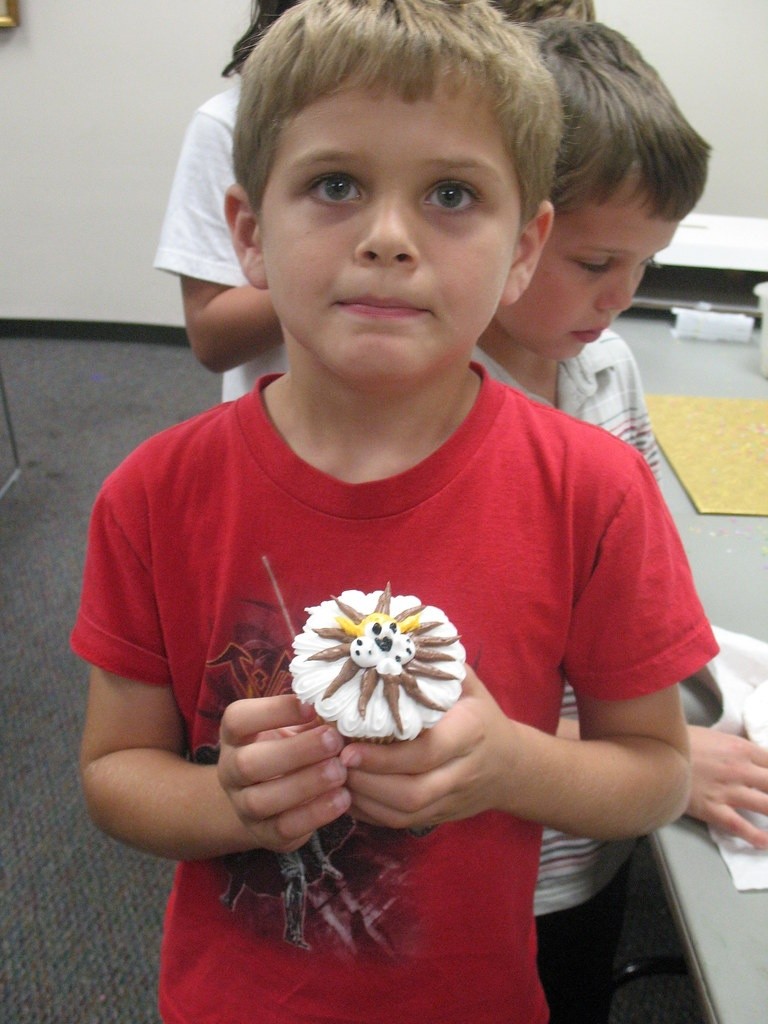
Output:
[601,315,768,1024]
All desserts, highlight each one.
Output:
[290,581,467,742]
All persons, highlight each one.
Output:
[70,0,721,1024]
[152,0,303,402]
[470,22,767,1022]
